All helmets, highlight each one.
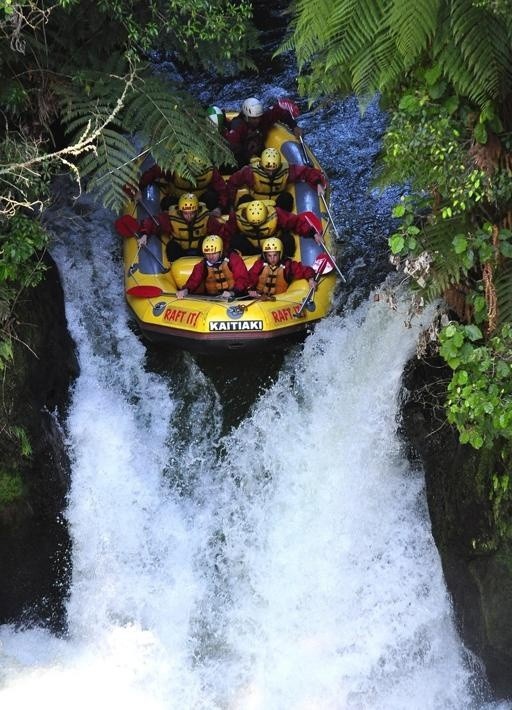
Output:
[262,237,283,260]
[202,235,223,256]
[187,150,208,168]
[178,193,198,212]
[246,201,266,225]
[243,98,266,118]
[261,148,281,170]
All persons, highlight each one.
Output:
[137,193,222,263]
[247,236,318,297]
[219,97,304,174]
[175,234,249,301]
[225,147,328,208]
[134,151,228,209]
[219,198,324,259]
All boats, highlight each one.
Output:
[118,109,341,349]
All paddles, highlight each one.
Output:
[123,184,162,227]
[303,211,347,281]
[127,287,238,303]
[115,214,168,273]
[293,254,337,317]
[310,177,342,242]
[277,97,312,165]
